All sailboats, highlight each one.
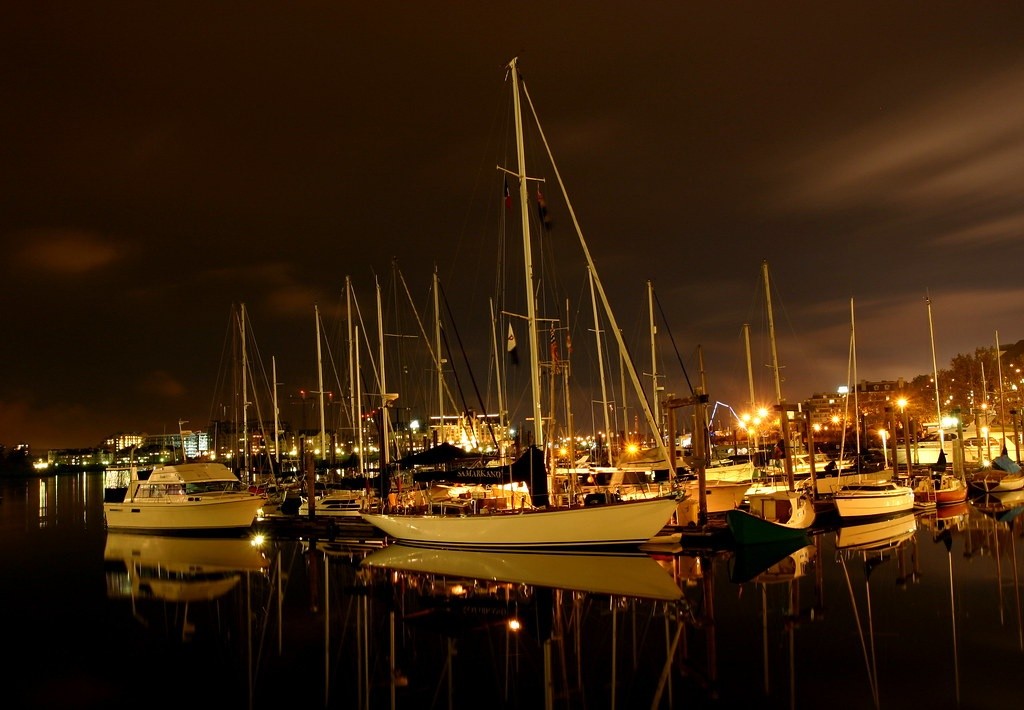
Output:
[99,54,1024,608]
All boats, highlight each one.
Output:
[357,541,687,605]
[101,532,272,607]
